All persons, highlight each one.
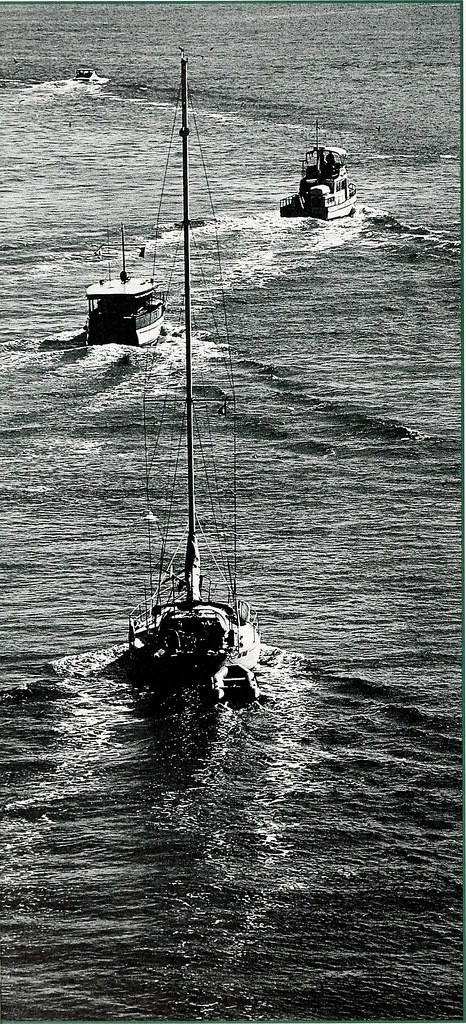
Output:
[326,152,337,166]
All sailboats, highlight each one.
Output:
[120,58,262,714]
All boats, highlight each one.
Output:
[73,67,111,87]
[279,119,359,221]
[83,223,168,346]
